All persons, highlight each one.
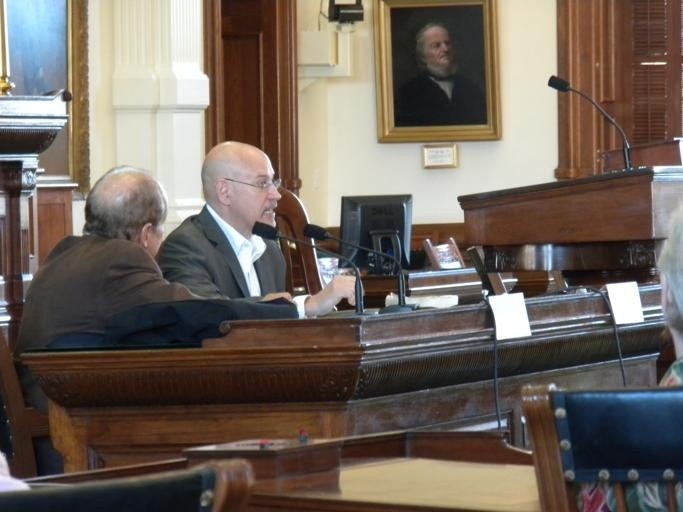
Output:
[13,165,365,350]
[569,201,683,512]
[394,20,481,126]
[151,138,288,301]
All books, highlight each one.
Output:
[384,291,461,311]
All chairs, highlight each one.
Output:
[521,384,683,510]
[0,460,254,511]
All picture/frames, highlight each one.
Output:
[372,0,506,143]
[421,143,460,170]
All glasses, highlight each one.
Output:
[220,176,284,190]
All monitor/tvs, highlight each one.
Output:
[339,196,411,277]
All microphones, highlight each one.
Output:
[251,221,366,317]
[548,75,632,171]
[304,223,415,314]
